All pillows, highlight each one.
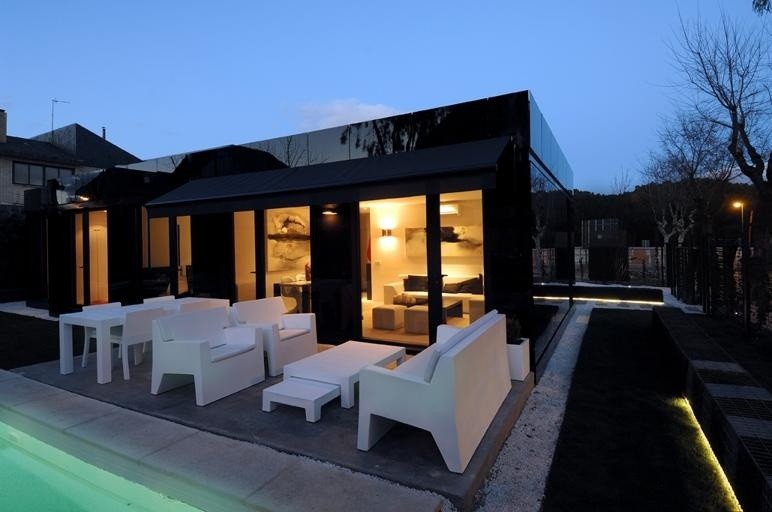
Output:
[401,274,484,295]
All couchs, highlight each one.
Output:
[381,273,484,316]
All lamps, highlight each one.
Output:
[375,207,401,253]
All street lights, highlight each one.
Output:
[732,200,747,236]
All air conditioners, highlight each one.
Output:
[438,203,462,217]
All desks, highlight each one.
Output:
[271,275,313,314]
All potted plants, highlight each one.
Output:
[500,312,533,382]
